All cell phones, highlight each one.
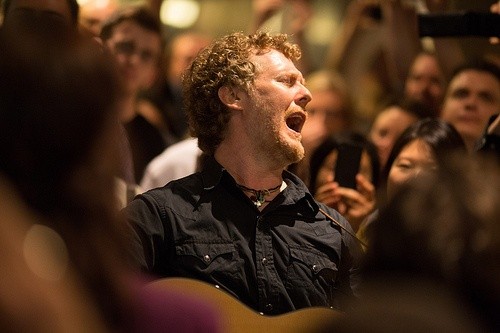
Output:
[335,146,361,189]
[417,11,500,39]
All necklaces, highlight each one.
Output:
[236,182,284,206]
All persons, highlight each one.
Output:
[118,30,367,316]
[80,0,222,208]
[285,46,500,290]
[0,12,217,333]
[0,0,79,28]
